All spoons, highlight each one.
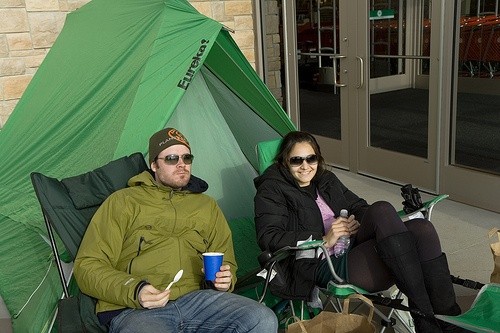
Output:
[165,269,184,291]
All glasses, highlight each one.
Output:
[287,153,319,167]
[157,154,194,165]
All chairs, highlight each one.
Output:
[29,140,499,333]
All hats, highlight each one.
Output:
[147,127,192,166]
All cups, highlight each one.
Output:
[202,252,224,280]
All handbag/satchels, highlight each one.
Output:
[284,294,374,333]
[487,227,500,284]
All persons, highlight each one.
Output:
[253,131,462,333]
[72,127,279,333]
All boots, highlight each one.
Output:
[376,231,443,333]
[421,252,466,333]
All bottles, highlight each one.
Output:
[334,209,350,258]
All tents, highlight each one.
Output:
[0,0,298,333]
